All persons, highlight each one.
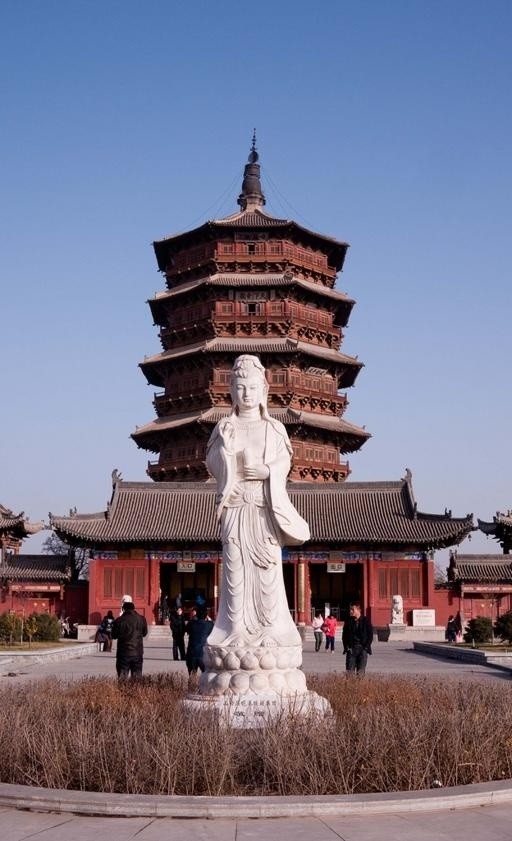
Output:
[113,602,147,682]
[312,613,324,652]
[162,595,170,624]
[170,607,187,661]
[447,616,458,642]
[191,608,199,621]
[341,602,373,678]
[186,606,216,675]
[176,593,183,609]
[97,623,110,651]
[321,614,337,654]
[201,356,315,667]
[58,613,78,641]
[454,612,463,642]
[104,611,116,648]
